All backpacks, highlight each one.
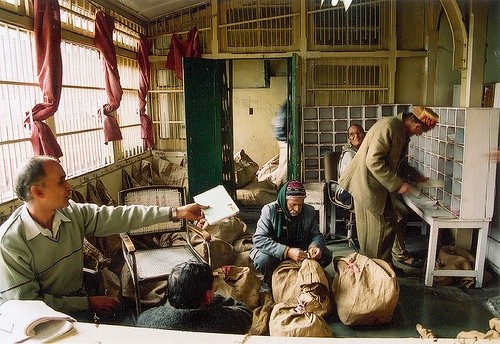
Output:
[271,98,291,141]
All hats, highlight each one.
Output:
[286,180,307,199]
[411,103,439,128]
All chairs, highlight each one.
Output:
[117,185,213,325]
[323,151,360,252]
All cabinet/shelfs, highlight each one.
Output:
[301,103,412,184]
[407,106,500,220]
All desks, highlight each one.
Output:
[402,191,490,287]
[301,182,327,235]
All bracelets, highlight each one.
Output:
[172,208,179,222]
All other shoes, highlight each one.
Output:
[391,265,405,277]
[399,257,425,269]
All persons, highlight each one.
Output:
[273,101,291,190]
[339,107,440,274]
[0,157,209,325]
[338,124,423,267]
[137,262,253,334]
[249,181,333,292]
